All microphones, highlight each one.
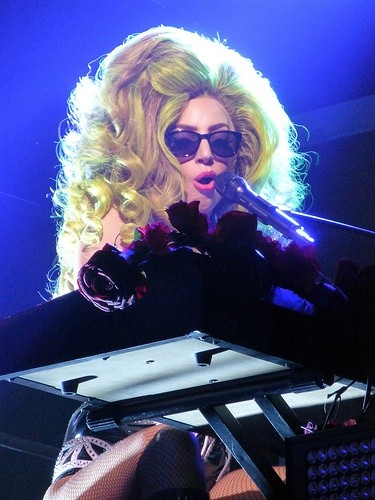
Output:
[216,172,315,247]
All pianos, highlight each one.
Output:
[1,238,374,435]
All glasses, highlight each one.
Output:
[163,130,243,158]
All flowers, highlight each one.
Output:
[78,200,320,312]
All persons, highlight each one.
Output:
[42,22,310,500]
